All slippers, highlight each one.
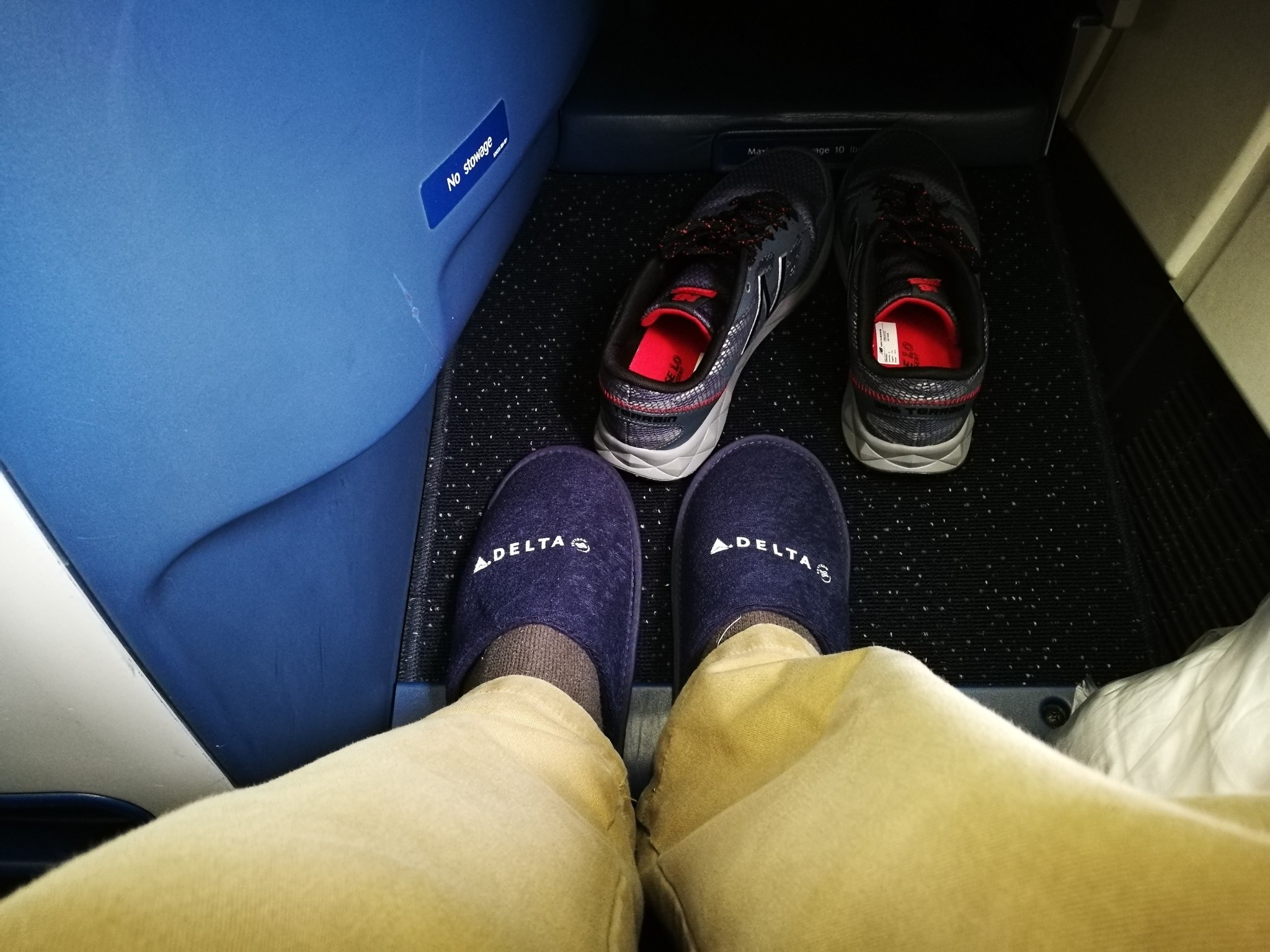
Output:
[670,432,852,745]
[441,446,642,774]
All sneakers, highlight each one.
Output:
[835,166,991,474]
[587,146,835,482]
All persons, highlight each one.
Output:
[0,433,1270,952]
